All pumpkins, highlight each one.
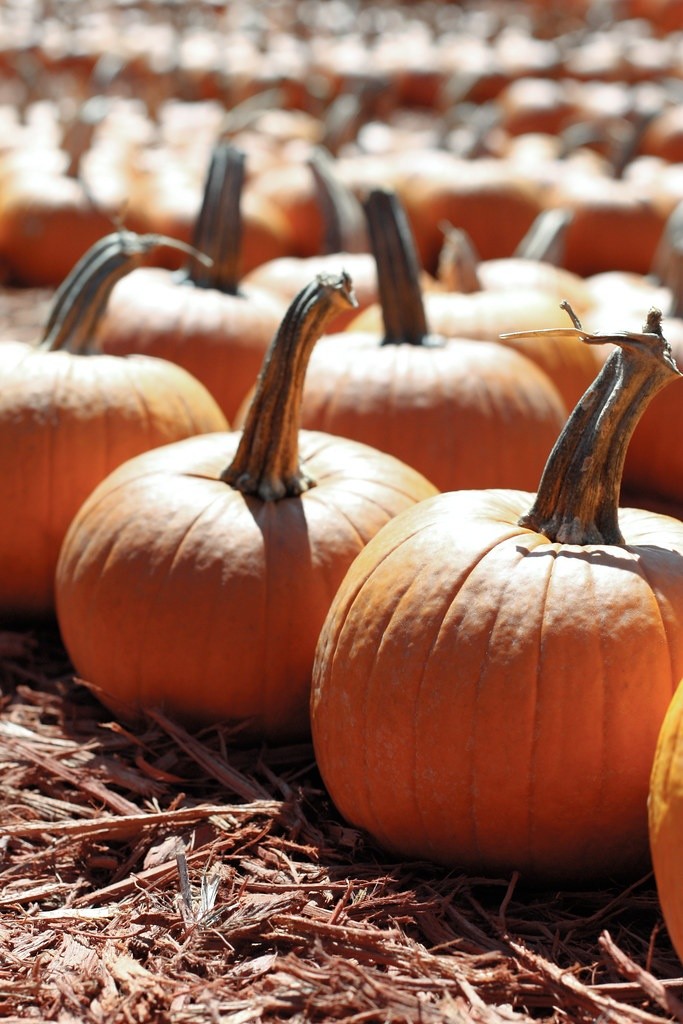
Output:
[0,0,683,964]
